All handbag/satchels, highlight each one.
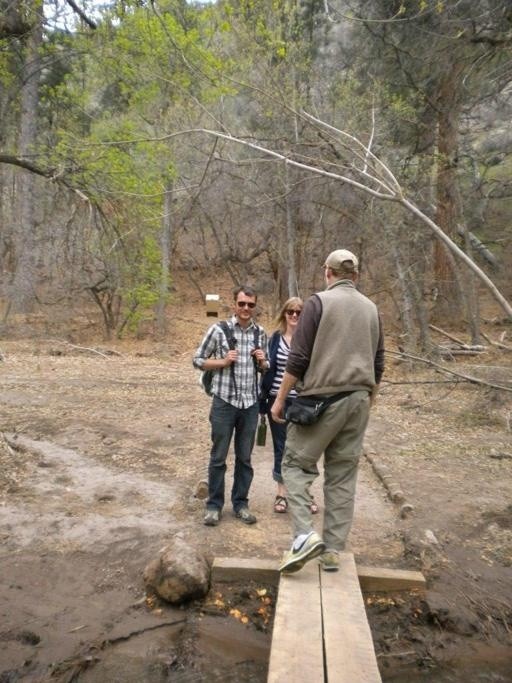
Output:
[285,398,327,426]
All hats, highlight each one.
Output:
[321,248,359,273]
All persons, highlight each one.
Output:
[192,285,272,525]
[256,296,319,515]
[270,249,385,574]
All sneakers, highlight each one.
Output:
[203,511,221,526]
[278,531,327,574]
[317,549,341,570]
[236,505,256,524]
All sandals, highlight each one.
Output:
[274,495,288,513]
[309,500,318,515]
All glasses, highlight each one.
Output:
[238,301,256,308]
[285,309,301,315]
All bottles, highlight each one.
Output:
[255,416,267,446]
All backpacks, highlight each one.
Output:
[203,322,259,394]
